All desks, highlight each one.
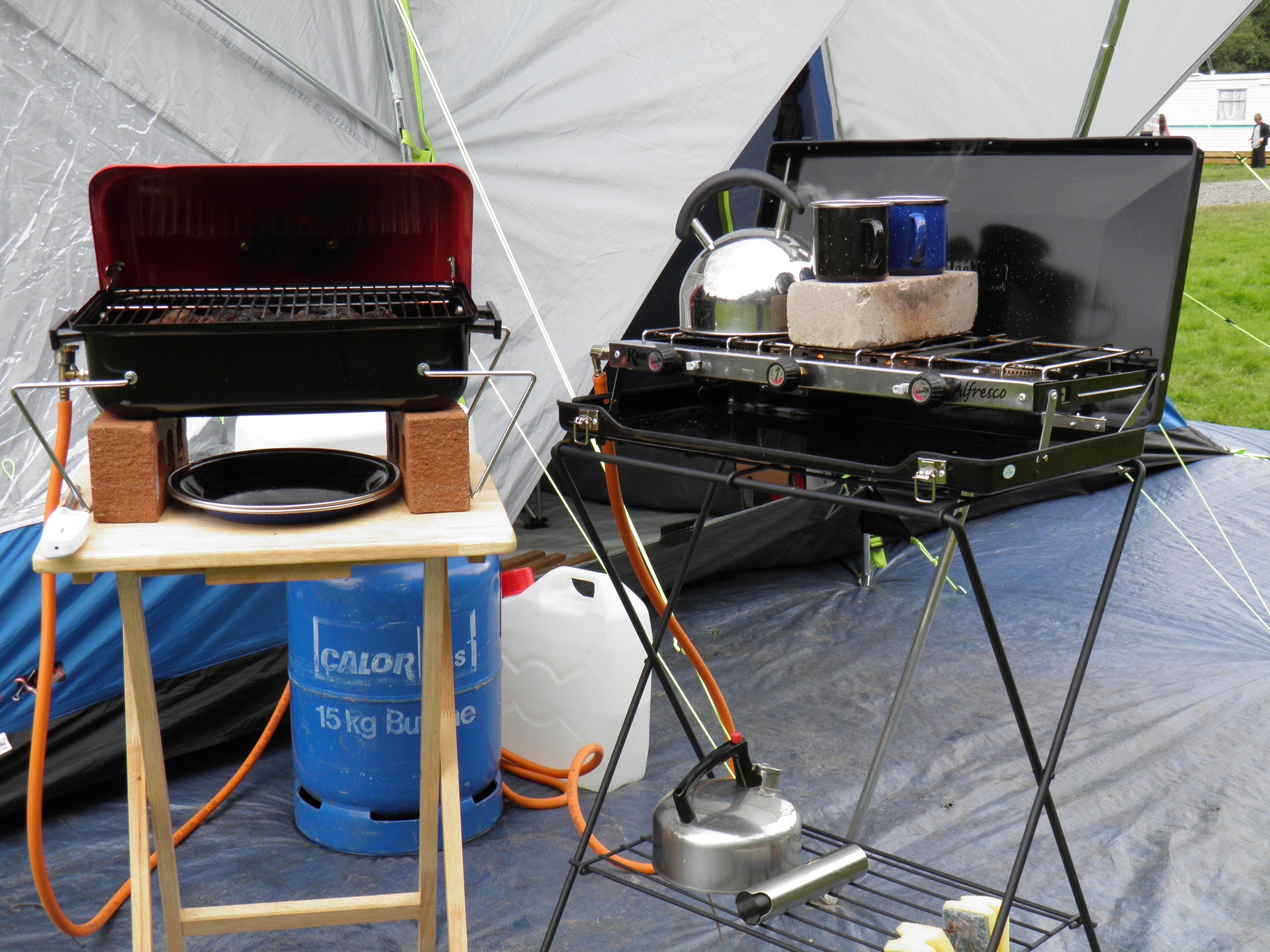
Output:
[33,454,517,952]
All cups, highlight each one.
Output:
[878,197,949,275]
[809,199,895,282]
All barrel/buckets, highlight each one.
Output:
[500,564,653,794]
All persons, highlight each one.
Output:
[1159,114,1170,137]
[1249,113,1270,168]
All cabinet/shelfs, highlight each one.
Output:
[538,434,1142,950]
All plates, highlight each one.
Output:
[165,448,401,526]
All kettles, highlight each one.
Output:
[674,167,817,336]
[650,734,803,896]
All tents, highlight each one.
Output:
[0,0,1270,832]
